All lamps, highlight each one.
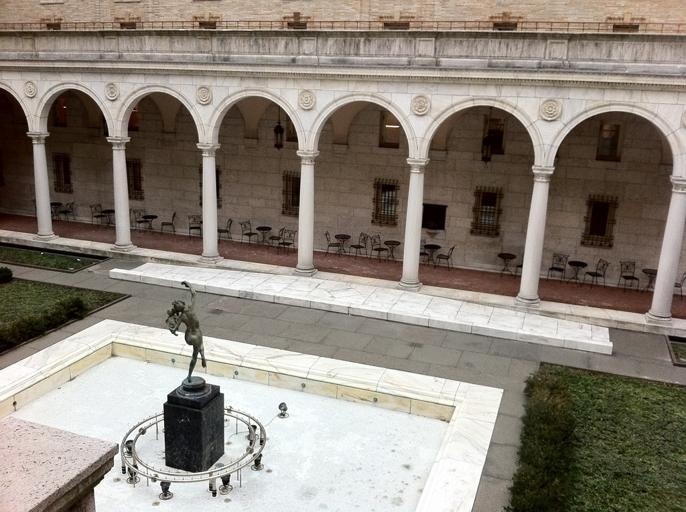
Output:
[272,114,285,153]
[479,106,495,165]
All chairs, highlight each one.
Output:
[519,250,684,295]
[48,198,117,231]
[215,214,295,256]
[129,205,201,238]
[418,240,456,270]
[321,230,401,263]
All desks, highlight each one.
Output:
[496,252,517,278]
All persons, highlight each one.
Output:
[169,280,206,383]
